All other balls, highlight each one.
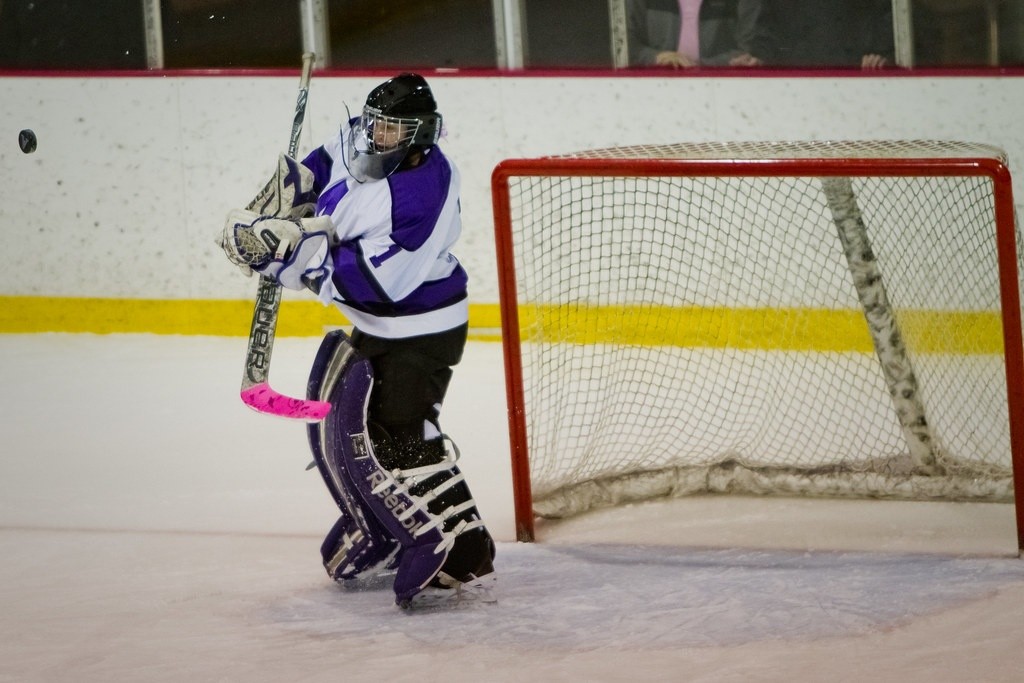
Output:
[18,129,37,154]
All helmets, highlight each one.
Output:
[361,72,443,154]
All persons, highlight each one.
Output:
[625,0,891,69]
[221,74,498,613]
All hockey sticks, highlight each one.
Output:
[239,50,333,425]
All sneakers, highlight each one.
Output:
[341,539,406,581]
[430,533,496,591]
